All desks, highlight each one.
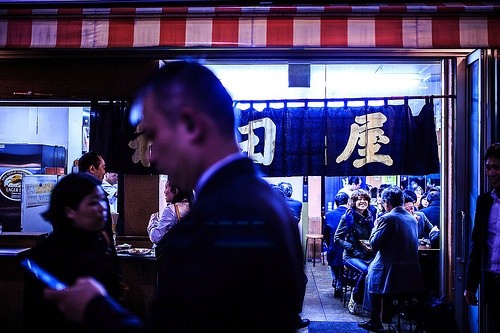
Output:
[358,239,441,253]
[112,247,164,263]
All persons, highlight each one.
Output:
[79,151,117,257]
[402,195,434,239]
[358,188,424,331]
[323,192,350,279]
[402,186,441,229]
[44,61,308,333]
[337,176,362,207]
[30,174,117,333]
[462,141,500,333]
[334,189,374,314]
[147,179,191,254]
[323,176,440,249]
[277,181,303,224]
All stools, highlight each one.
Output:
[340,264,359,310]
[305,234,325,267]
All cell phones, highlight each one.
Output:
[21,258,68,291]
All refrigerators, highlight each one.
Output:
[0,143,68,233]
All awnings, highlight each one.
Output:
[0,6,500,49]
